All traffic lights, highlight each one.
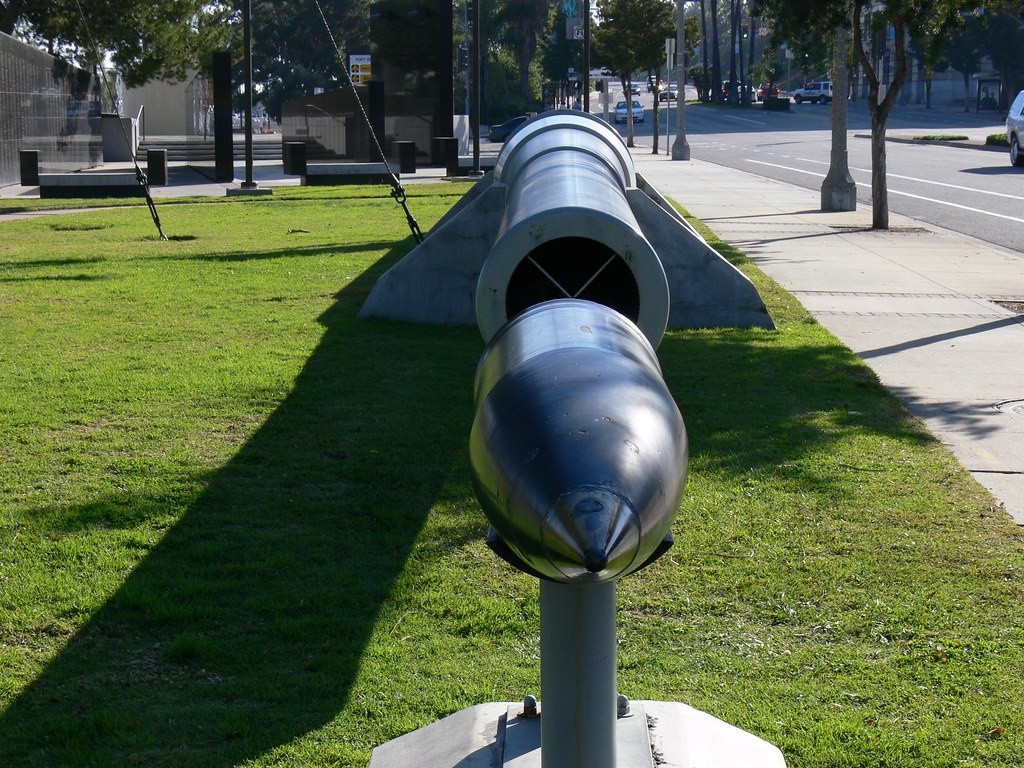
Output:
[742,26,749,39]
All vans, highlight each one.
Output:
[600,66,614,77]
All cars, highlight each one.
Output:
[646,75,662,93]
[233,109,268,130]
[1005,90,1024,167]
[612,100,645,123]
[658,85,678,103]
[738,85,757,102]
[624,84,641,95]
[757,82,778,101]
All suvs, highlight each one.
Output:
[722,80,742,99]
[793,80,834,105]
